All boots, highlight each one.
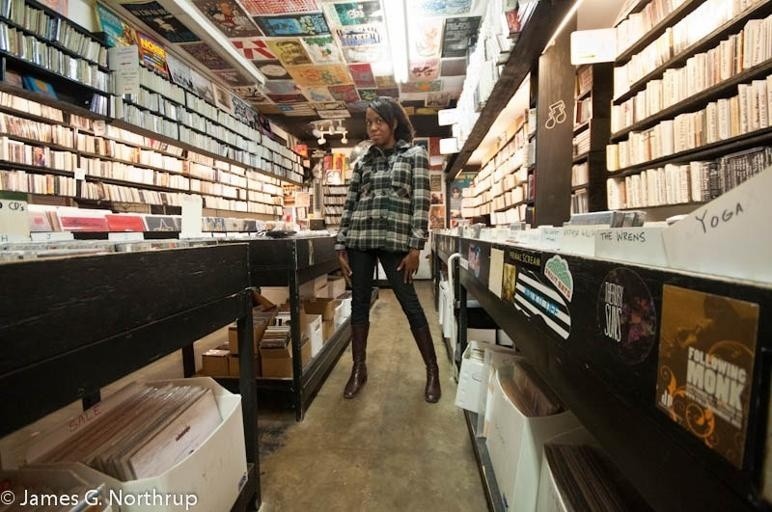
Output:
[412,324,441,402]
[345,323,370,397]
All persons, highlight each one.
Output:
[332,96,442,404]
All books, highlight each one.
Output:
[1,1,351,511]
[427,1,772,511]
